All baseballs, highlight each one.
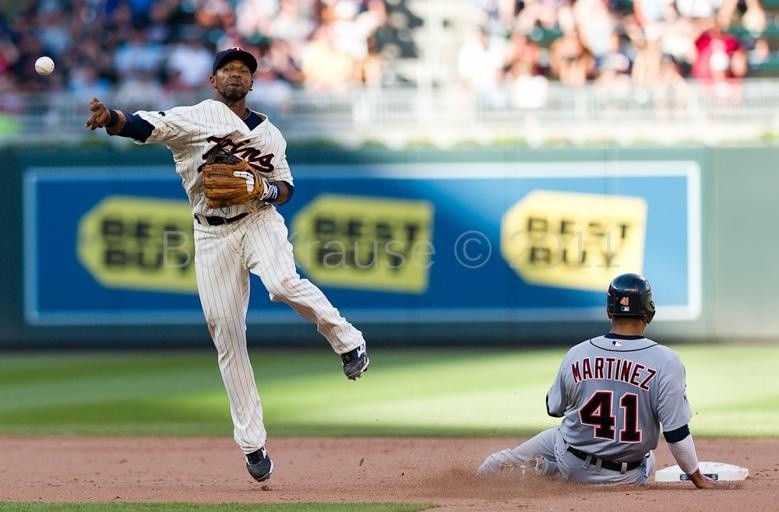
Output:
[35,56,56,77]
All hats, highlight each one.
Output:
[211,46,259,77]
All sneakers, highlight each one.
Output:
[524,454,558,479]
[340,334,370,380]
[243,444,275,482]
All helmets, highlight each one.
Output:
[606,272,656,318]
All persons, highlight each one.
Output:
[84,46,369,481]
[477,274,722,488]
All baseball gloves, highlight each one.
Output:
[202,151,273,208]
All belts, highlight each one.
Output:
[193,210,248,226]
[563,438,645,472]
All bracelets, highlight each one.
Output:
[106,108,119,128]
